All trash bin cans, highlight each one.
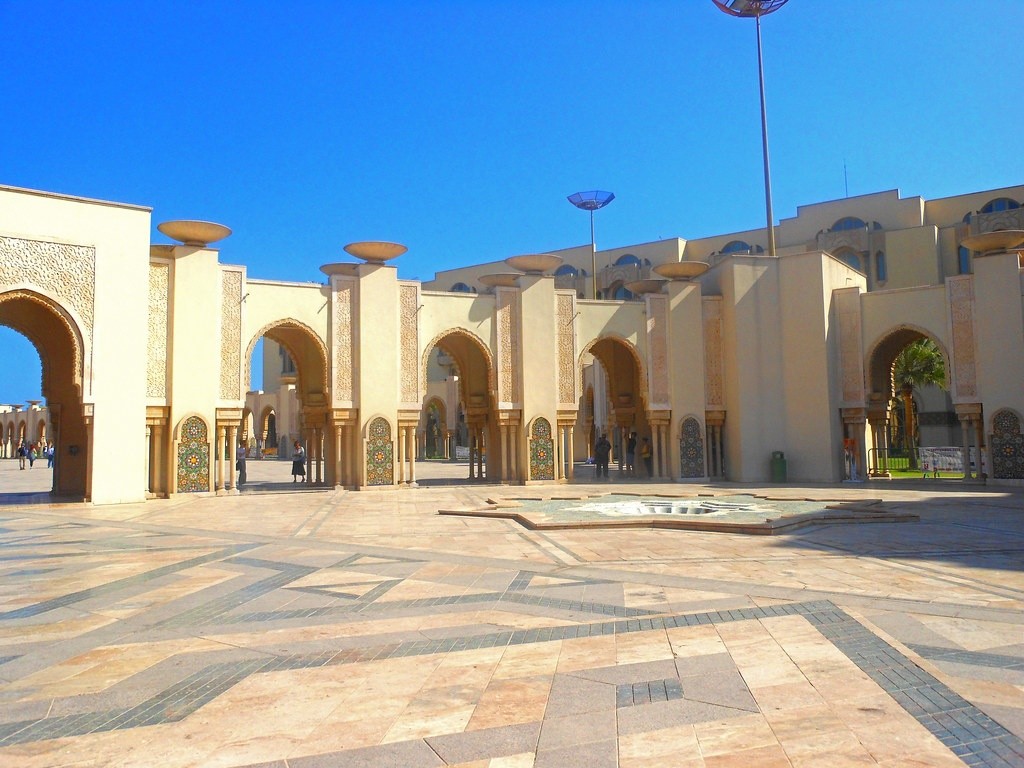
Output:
[770,451,787,483]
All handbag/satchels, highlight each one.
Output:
[301,458,305,463]
[236,462,240,470]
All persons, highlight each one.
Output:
[594,433,611,480]
[18,442,26,470]
[237,440,247,486]
[28,445,36,469]
[47,445,54,468]
[922,466,928,479]
[291,440,306,483]
[625,432,636,476]
[640,437,653,480]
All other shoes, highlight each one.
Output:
[302,479,305,482]
[293,480,296,483]
